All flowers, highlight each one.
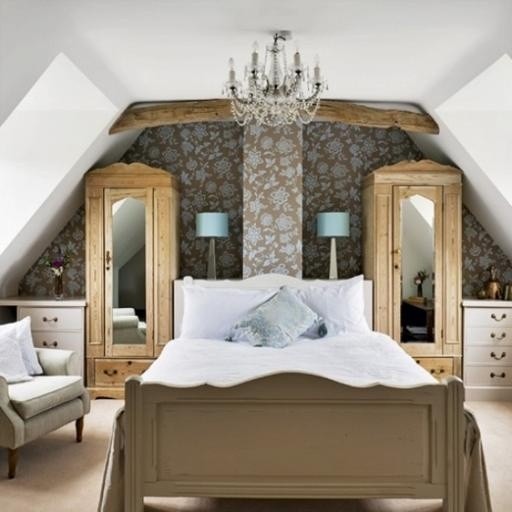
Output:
[413,268,430,286]
[34,243,73,278]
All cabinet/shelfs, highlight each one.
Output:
[0,294,86,384]
[361,158,464,384]
[83,160,179,404]
[460,296,512,403]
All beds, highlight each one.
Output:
[96,270,498,512]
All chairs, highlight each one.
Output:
[0,347,92,480]
[111,305,143,344]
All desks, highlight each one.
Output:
[402,299,435,343]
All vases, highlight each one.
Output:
[53,274,65,300]
[416,284,423,297]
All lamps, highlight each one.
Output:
[193,211,230,282]
[315,211,351,280]
[217,27,330,131]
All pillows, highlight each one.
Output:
[229,283,323,349]
[179,282,298,341]
[1,323,35,382]
[284,273,372,335]
[0,314,47,376]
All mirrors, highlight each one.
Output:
[391,184,444,357]
[103,186,156,358]
